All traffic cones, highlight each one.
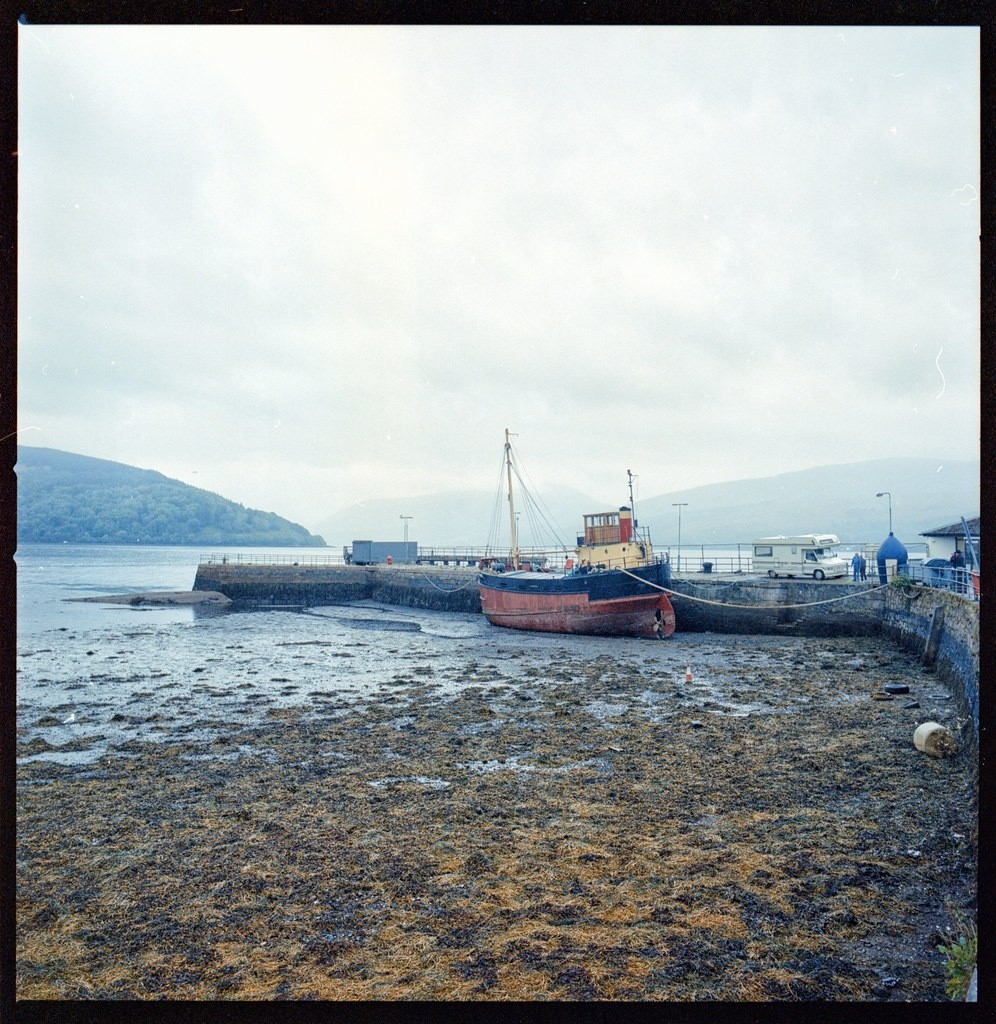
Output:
[686,663,693,681]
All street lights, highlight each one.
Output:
[672,503,688,572]
[876,492,891,532]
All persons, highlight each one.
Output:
[851,553,867,583]
[950,550,964,575]
[564,555,605,577]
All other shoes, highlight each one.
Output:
[865,579,867,581]
[861,579,863,581]
[852,579,859,581]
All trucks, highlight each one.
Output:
[751,534,849,581]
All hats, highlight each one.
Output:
[954,549,963,554]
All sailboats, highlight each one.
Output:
[476,427,677,640]
